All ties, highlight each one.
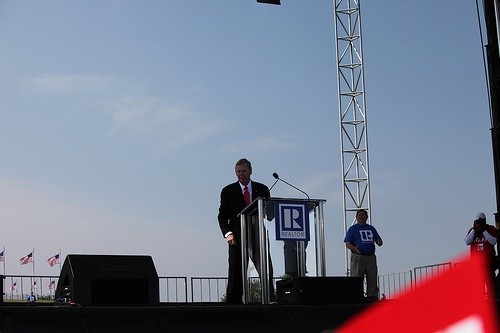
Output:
[243,187,250,206]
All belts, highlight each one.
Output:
[354,252,375,256]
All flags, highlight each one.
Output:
[10,283,16,290]
[0,252,4,262]
[47,254,59,267]
[48,281,55,289]
[20,253,32,264]
[30,282,36,289]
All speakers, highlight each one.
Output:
[275,276,364,304]
[54,254,160,306]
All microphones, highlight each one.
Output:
[272,173,310,199]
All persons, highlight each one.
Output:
[464,212,498,274]
[344,210,383,300]
[218,158,275,302]
[27,292,36,303]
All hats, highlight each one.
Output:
[476,212,486,219]
